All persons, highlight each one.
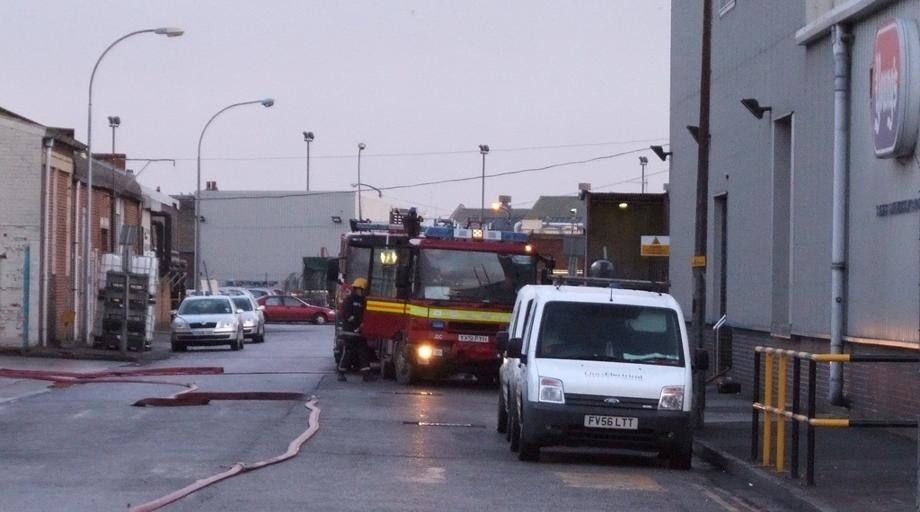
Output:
[335,278,377,382]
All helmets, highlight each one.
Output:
[351,277,369,290]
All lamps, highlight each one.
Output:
[740,97,772,120]
[650,145,673,163]
[685,125,712,145]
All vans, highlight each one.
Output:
[497,275,693,469]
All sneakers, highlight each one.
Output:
[336,369,347,382]
[362,372,378,382]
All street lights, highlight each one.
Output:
[480,143,490,223]
[86,27,185,348]
[357,143,366,220]
[195,98,275,295]
[638,156,649,192]
[303,130,314,192]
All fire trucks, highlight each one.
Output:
[334,217,556,385]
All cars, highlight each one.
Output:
[169,284,337,352]
